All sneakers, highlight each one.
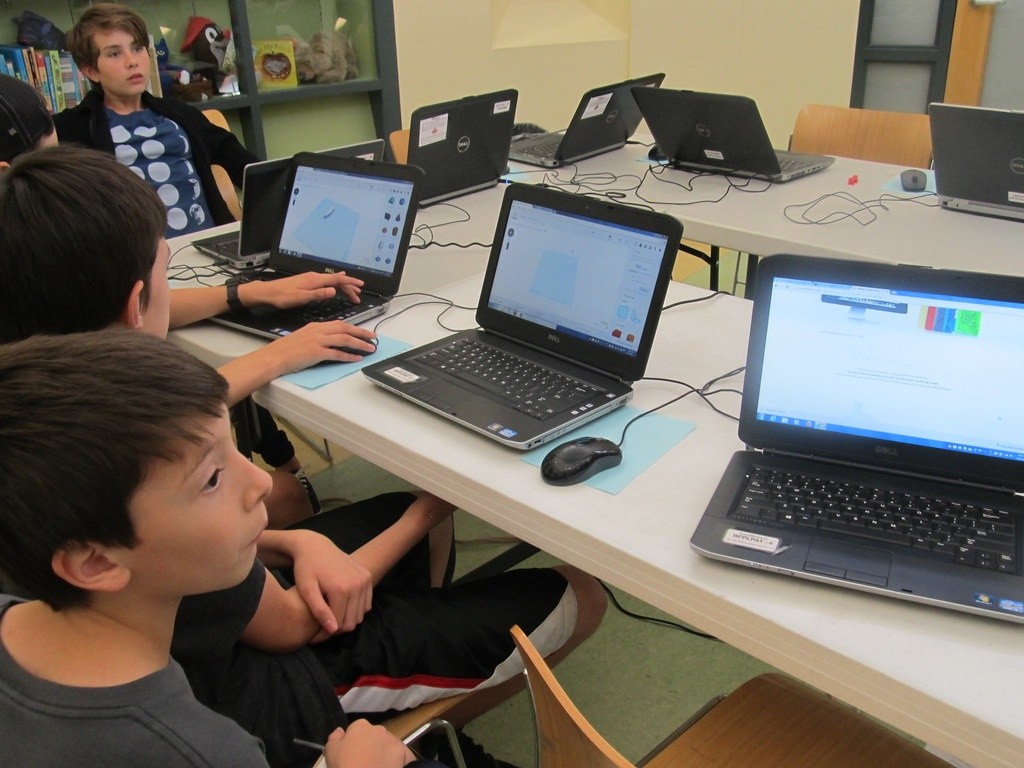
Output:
[287,466,323,515]
[416,732,519,768]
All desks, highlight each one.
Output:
[164,129,1024,768]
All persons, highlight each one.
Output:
[0,74,59,169]
[0,329,425,768]
[52,4,265,239]
[0,143,609,768]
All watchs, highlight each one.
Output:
[224,276,249,313]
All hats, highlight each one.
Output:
[0,72,53,162]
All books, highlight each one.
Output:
[0,47,91,115]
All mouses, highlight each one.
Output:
[900,169,927,191]
[317,336,377,367]
[541,436,622,486]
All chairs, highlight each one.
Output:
[380,689,475,768]
[788,103,934,172]
[511,624,957,768]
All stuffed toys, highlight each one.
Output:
[12,10,65,51]
[155,14,239,104]
[285,28,360,84]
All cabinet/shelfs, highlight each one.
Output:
[0,0,403,164]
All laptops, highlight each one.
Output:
[690,253,1024,626]
[508,73,666,168]
[929,102,1024,221]
[190,139,385,269]
[630,87,835,183]
[362,183,684,451]
[406,89,519,209]
[206,152,420,341]
